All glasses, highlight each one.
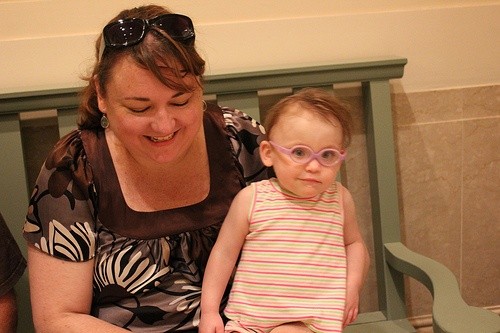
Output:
[98,13,196,63]
[268,140,349,167]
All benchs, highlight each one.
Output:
[0,58,500,333]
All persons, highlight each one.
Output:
[198,87,365,333]
[22,4,370,333]
[0,212,27,333]
